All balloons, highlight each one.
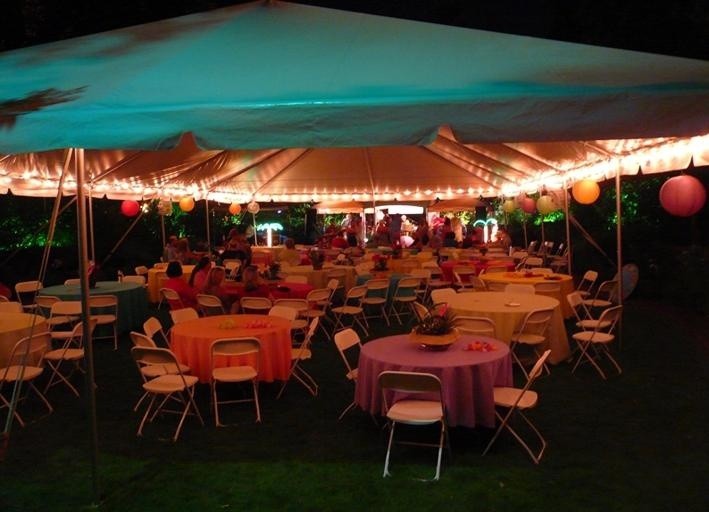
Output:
[658,174,706,218]
[121,200,139,217]
[247,202,259,214]
[486,206,492,211]
[502,200,515,213]
[179,196,194,211]
[536,195,556,215]
[571,178,601,204]
[229,203,241,215]
[519,197,536,213]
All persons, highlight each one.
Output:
[500,229,511,244]
[190,257,211,292]
[332,231,349,248]
[412,217,429,244]
[327,218,337,233]
[232,247,257,286]
[279,239,297,262]
[341,214,352,227]
[441,218,455,247]
[197,265,240,315]
[228,228,240,247]
[166,236,183,261]
[165,261,199,314]
[374,213,393,234]
[430,229,441,244]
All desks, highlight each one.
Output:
[442,260,515,281]
[0,312,47,367]
[252,245,283,256]
[228,282,313,298]
[357,335,512,428]
[287,263,357,288]
[438,292,568,365]
[480,271,574,320]
[171,313,291,385]
[39,280,147,337]
[148,265,195,303]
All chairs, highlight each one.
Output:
[269,307,296,325]
[504,284,535,295]
[422,266,451,287]
[160,288,186,308]
[332,285,369,337]
[196,295,226,316]
[510,307,554,380]
[334,328,377,424]
[377,372,450,482]
[486,266,506,273]
[568,292,613,360]
[224,259,240,269]
[144,317,171,347]
[171,307,197,324]
[0,302,24,313]
[47,302,82,339]
[359,279,390,327]
[411,269,432,302]
[525,258,542,267]
[573,305,623,380]
[327,279,338,305]
[388,277,422,325]
[414,303,431,324]
[65,279,80,286]
[130,332,190,410]
[483,349,552,463]
[547,244,569,272]
[276,317,319,399]
[90,296,119,350]
[528,241,553,257]
[454,315,496,336]
[209,338,262,426]
[118,272,126,283]
[454,266,475,273]
[582,281,617,319]
[37,296,62,316]
[43,318,97,399]
[533,281,563,298]
[576,271,598,299]
[308,288,331,339]
[136,266,147,275]
[15,281,43,307]
[468,275,487,292]
[132,346,205,442]
[273,298,309,336]
[452,272,470,293]
[0,332,52,427]
[241,297,272,313]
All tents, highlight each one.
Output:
[0,5,709,198]
